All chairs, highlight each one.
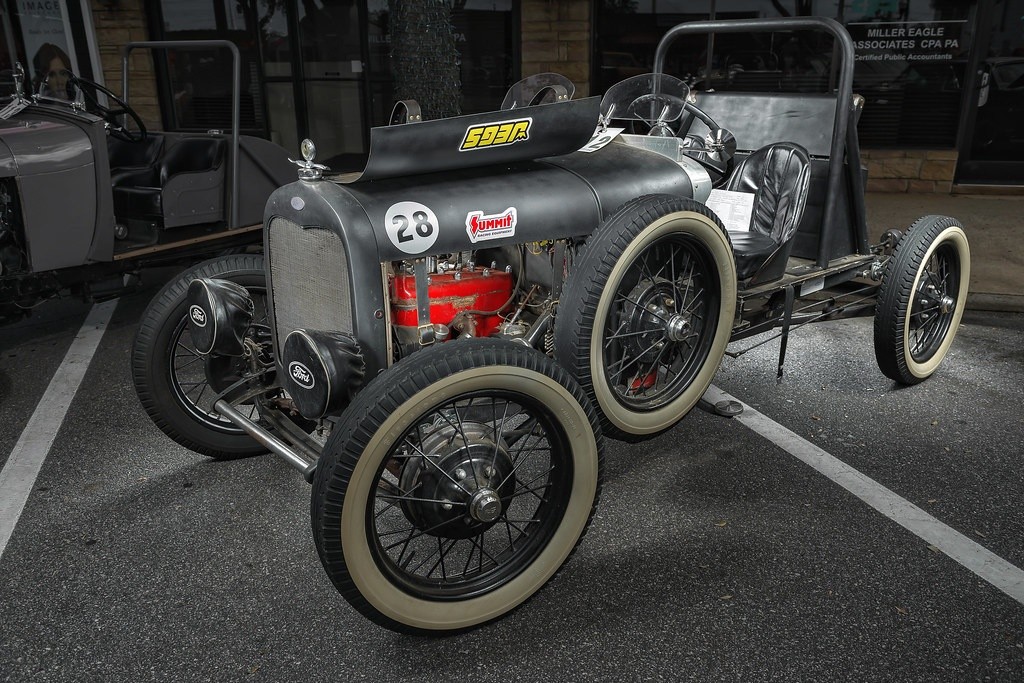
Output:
[682,134,733,188]
[106,131,166,186]
[705,139,811,287]
[109,135,228,231]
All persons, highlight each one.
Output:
[31,42,76,104]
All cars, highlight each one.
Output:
[0,39,311,326]
[131,18,971,635]
